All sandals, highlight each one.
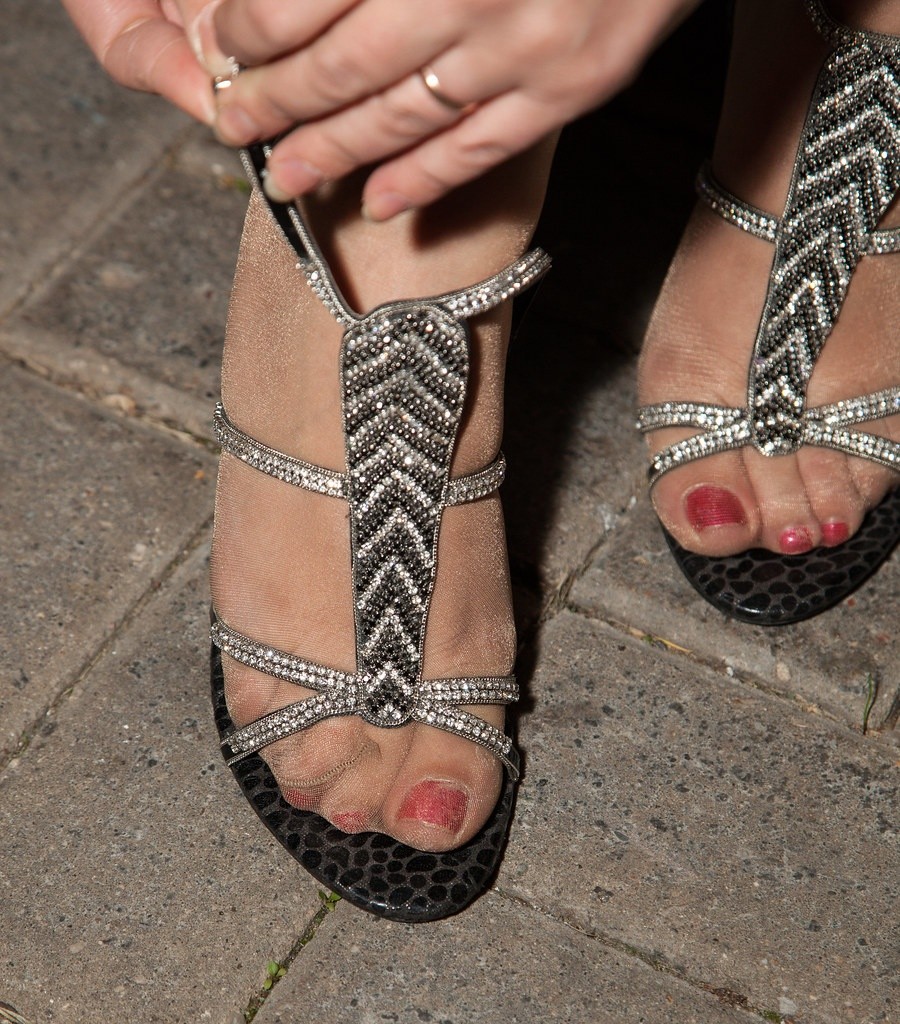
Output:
[208,142,555,922]
[635,0,899,628]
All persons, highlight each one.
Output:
[67,0,900,922]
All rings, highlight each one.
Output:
[421,66,463,108]
